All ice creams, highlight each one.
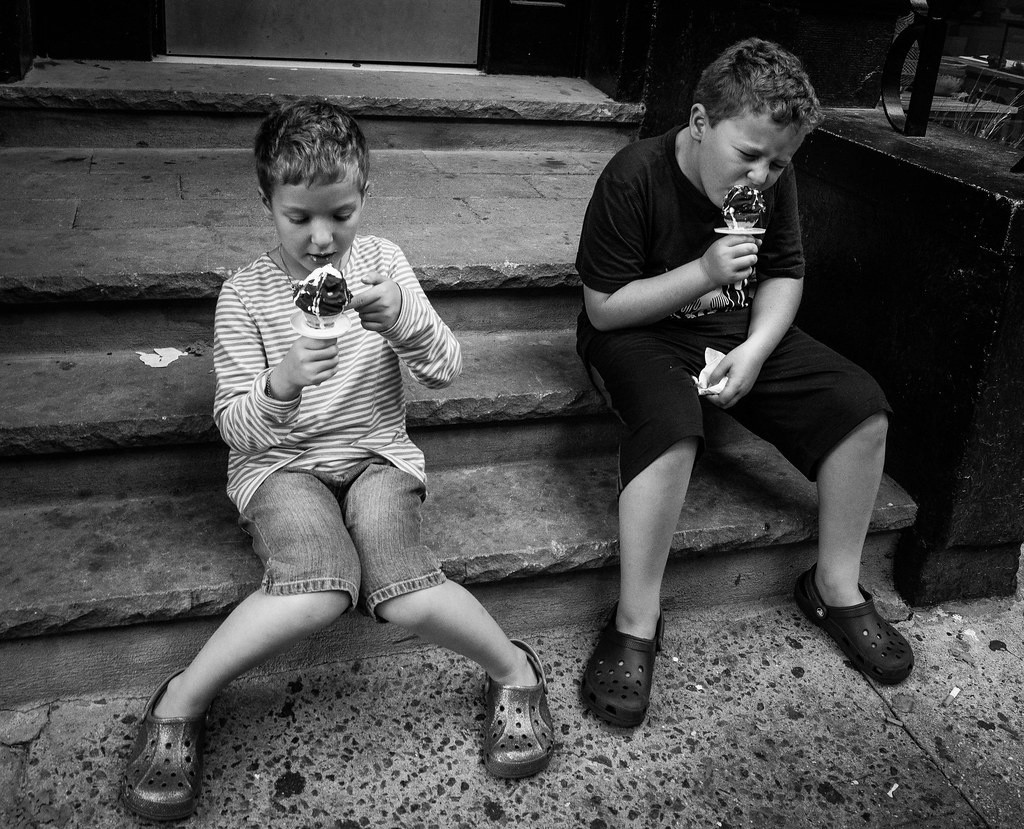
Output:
[291,263,354,330]
[720,185,767,230]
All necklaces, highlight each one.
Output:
[277,243,344,290]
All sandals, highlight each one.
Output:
[582,599,665,729]
[795,561,914,684]
[485,638,556,779]
[125,665,213,819]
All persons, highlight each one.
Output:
[117,100,556,824]
[570,40,917,731]
